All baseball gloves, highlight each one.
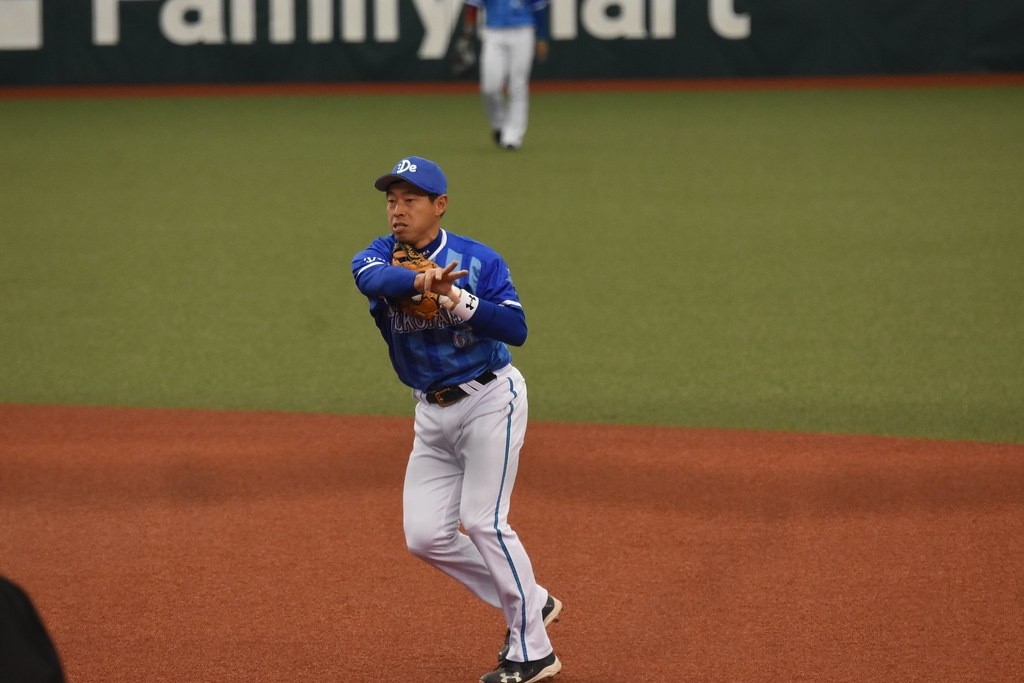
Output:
[393,242,442,320]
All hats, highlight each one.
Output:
[374,155,448,198]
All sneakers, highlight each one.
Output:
[478,648,562,683]
[498,591,562,664]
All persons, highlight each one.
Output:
[353,149,569,683]
[462,0,553,155]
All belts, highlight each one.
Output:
[426,371,497,407]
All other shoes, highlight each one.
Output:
[491,127,521,149]
